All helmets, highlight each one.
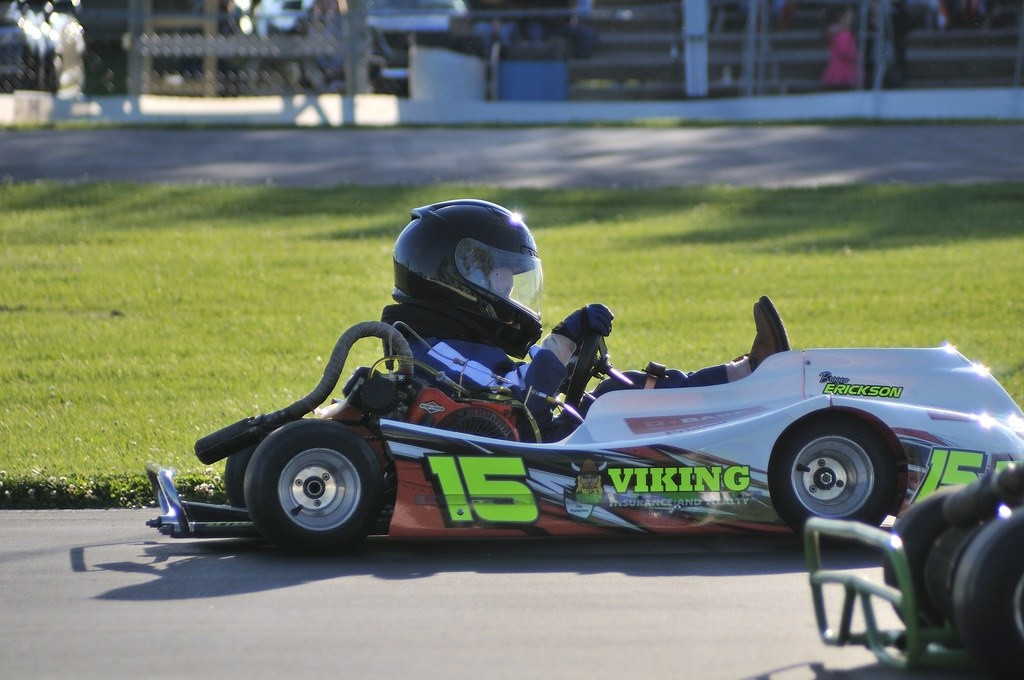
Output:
[392,198,544,360]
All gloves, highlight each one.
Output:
[551,303,614,345]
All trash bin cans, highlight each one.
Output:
[405,29,490,104]
[489,41,574,103]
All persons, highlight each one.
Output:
[75,0,128,95]
[219,1,392,95]
[380,198,790,443]
[821,4,859,89]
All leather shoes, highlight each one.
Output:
[747,295,792,374]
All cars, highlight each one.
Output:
[59,0,242,96]
[0,0,72,95]
[250,1,468,98]
